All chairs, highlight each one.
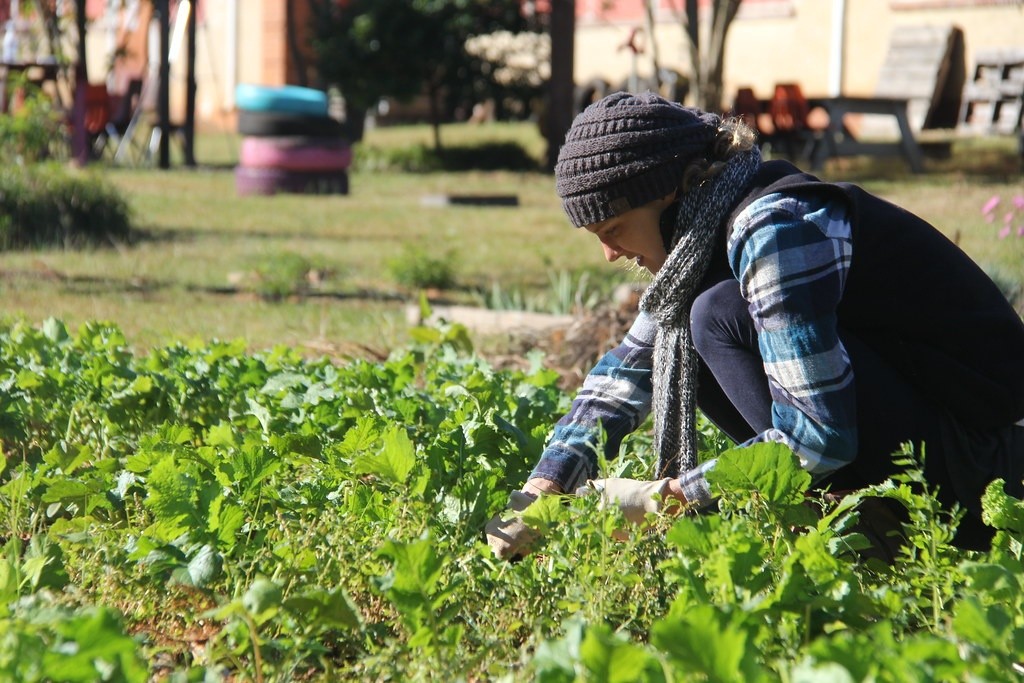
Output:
[735,89,771,153]
[771,83,836,166]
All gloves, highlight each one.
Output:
[485,490,534,558]
[577,477,672,541]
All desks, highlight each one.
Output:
[754,96,925,175]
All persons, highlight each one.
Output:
[484,92,1024,560]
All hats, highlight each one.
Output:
[555,91,719,228]
